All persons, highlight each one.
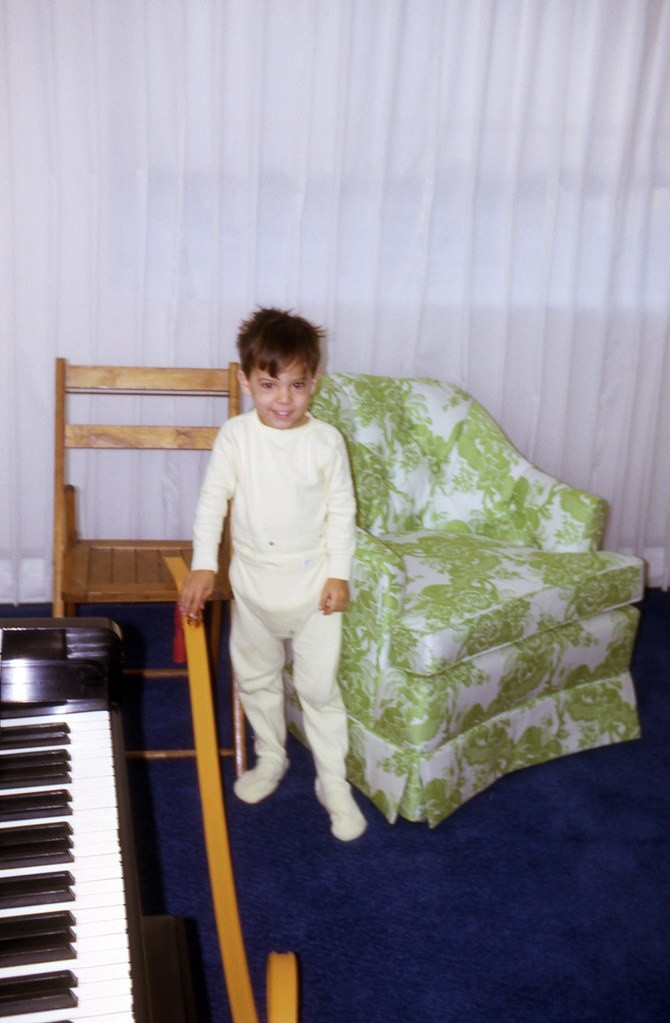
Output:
[177,308,367,842]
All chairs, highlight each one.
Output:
[53,356,248,780]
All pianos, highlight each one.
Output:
[2,608,209,1022]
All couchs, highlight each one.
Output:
[282,371,646,827]
